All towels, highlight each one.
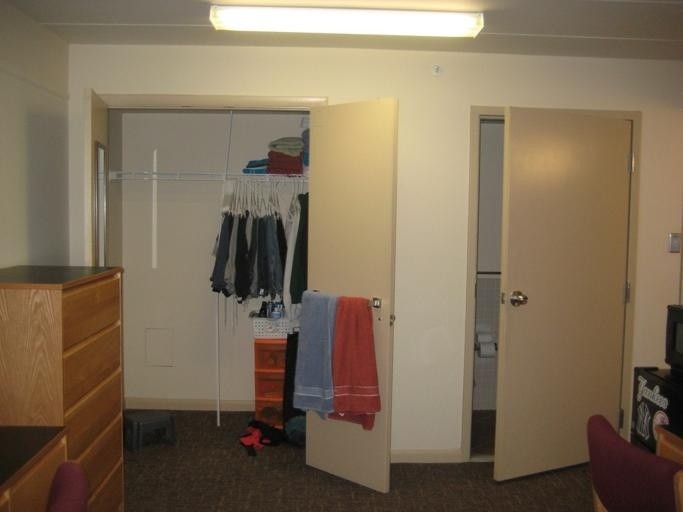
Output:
[293,292,381,431]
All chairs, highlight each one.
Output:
[587,415,683,512]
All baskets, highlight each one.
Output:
[252,309,289,340]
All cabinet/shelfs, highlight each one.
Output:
[254,339,288,425]
[0,266,125,512]
[630,366,683,454]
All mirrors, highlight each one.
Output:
[93,141,108,267]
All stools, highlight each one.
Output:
[124,412,177,452]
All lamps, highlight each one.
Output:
[209,5,485,39]
